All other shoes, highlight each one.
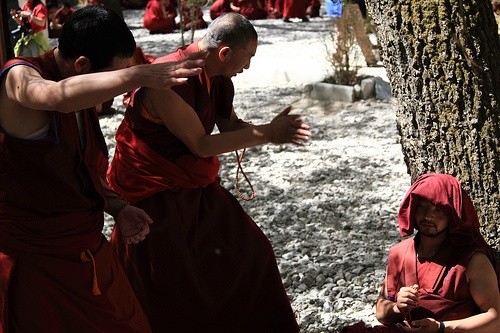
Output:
[99,108,115,114]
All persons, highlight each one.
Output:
[0,5,210,333]
[0,0,382,67]
[106,12,312,333]
[375,172,500,333]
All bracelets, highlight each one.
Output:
[438,320,445,333]
